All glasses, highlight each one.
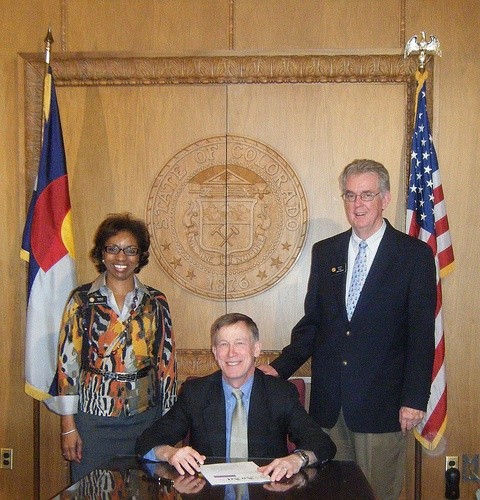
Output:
[341,190,382,202]
[103,245,142,256]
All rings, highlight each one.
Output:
[413,424,417,427]
[284,468,287,471]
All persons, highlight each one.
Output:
[145,460,328,500]
[254,159,437,500]
[136,312,338,482]
[44,214,178,482]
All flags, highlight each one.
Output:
[405,71,456,449]
[19,63,79,403]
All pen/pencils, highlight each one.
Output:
[141,474,175,484]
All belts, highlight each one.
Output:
[84,364,153,382]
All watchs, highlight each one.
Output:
[294,450,309,466]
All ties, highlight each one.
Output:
[232,483,245,500]
[346,240,368,322]
[230,388,249,458]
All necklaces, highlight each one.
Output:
[90,289,139,357]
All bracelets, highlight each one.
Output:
[62,429,76,435]
[301,469,309,481]
